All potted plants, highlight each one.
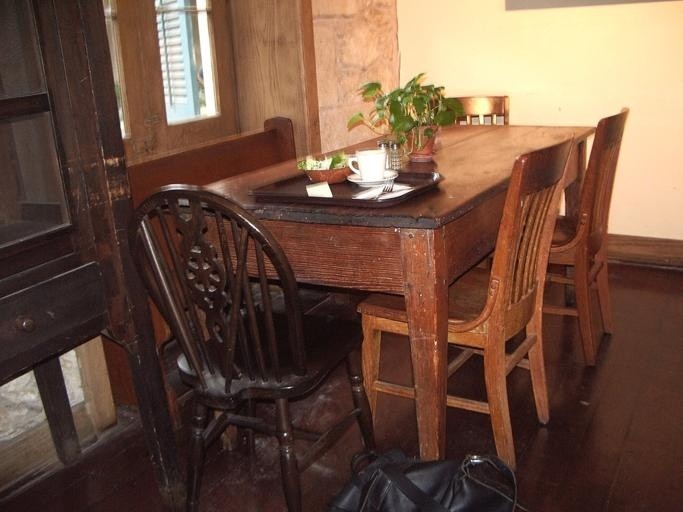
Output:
[349,72,459,156]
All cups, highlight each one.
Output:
[348,149,386,180]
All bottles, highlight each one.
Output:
[392,143,401,170]
[378,143,391,169]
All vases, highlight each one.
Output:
[407,124,435,165]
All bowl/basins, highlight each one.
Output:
[295,158,350,185]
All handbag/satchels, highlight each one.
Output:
[329,450,519,512]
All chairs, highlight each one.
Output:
[354,128,579,469]
[124,183,382,511]
[428,95,513,128]
[496,102,631,367]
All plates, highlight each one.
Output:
[344,171,397,187]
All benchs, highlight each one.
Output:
[95,117,328,455]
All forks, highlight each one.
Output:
[369,179,395,200]
